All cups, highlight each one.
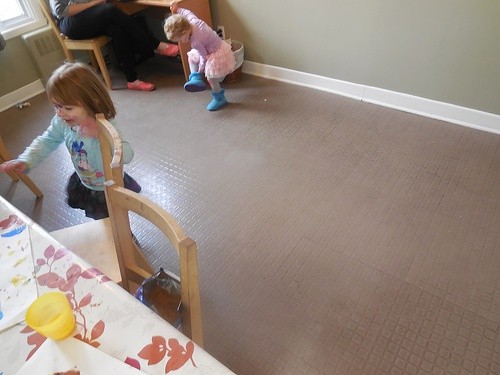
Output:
[25,291,76,339]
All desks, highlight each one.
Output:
[0,191,239,375]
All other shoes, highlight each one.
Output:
[127,79,156,91]
[153,41,181,57]
[184,72,207,92]
[207,88,227,111]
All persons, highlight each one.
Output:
[1,62,141,246]
[163,2,236,112]
[50,0,181,91]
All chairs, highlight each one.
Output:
[38,0,119,89]
[102,180,205,345]
[48,111,122,285]
